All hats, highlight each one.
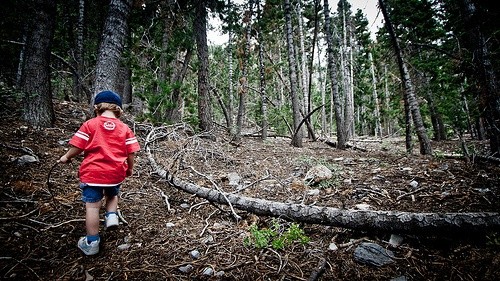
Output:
[94,90,122,109]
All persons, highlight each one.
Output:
[59,90,141,254]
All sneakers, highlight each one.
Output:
[104,214,119,232]
[77,235,100,255]
